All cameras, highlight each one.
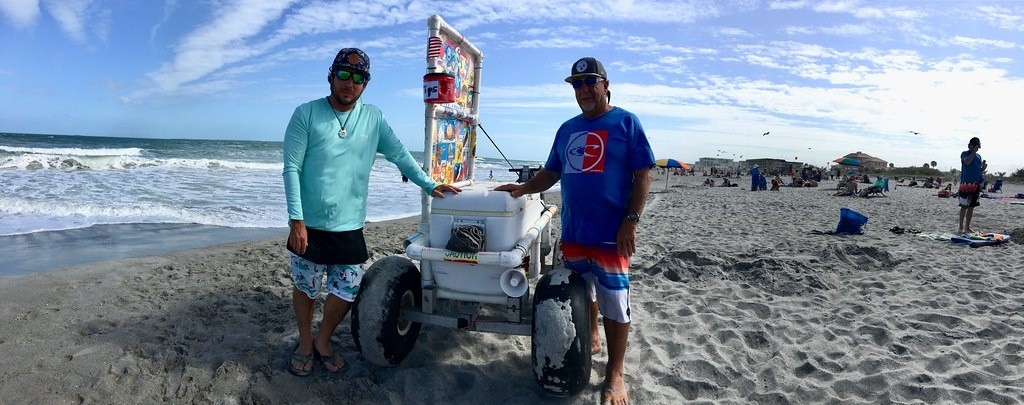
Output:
[983,160,986,164]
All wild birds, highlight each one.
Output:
[808,148,811,149]
[763,132,770,136]
[795,157,798,160]
[716,150,744,159]
[909,131,922,136]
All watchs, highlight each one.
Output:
[625,211,640,223]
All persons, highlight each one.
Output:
[283,48,462,376]
[829,169,885,197]
[952,138,988,234]
[746,163,834,191]
[701,165,743,187]
[401,172,408,182]
[489,171,493,179]
[494,57,657,405]
[658,165,695,176]
[894,175,958,198]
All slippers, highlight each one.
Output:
[313,338,345,373]
[890,226,905,234]
[290,343,313,376]
[910,227,920,233]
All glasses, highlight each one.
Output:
[332,69,366,84]
[572,76,605,88]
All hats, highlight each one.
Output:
[564,57,607,83]
[332,48,371,81]
[968,137,981,148]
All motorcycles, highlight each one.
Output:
[351,165,594,399]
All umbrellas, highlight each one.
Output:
[834,158,865,175]
[655,158,690,189]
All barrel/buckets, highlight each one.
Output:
[424,73,455,103]
[837,208,868,234]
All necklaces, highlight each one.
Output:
[328,97,357,138]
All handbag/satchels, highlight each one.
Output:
[835,208,868,234]
